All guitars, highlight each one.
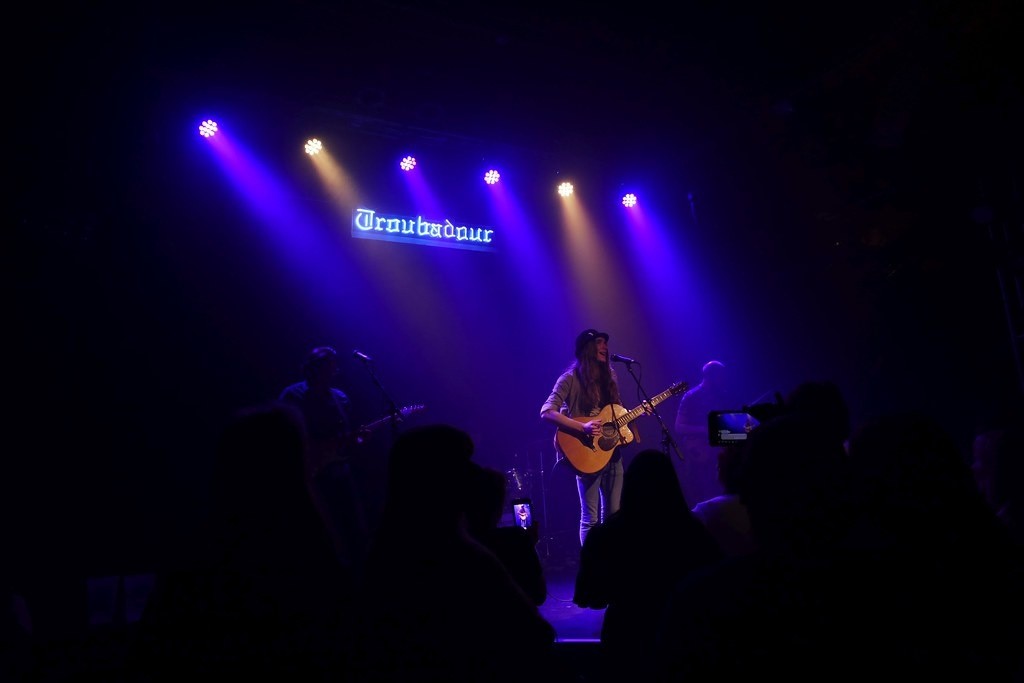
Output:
[309,399,425,489]
[682,389,772,466]
[553,380,688,478]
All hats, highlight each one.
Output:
[575,328,609,356]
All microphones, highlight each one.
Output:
[610,354,638,365]
[352,350,371,361]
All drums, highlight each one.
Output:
[502,466,539,503]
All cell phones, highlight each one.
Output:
[708,410,762,447]
[512,497,533,531]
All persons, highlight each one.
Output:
[743,414,757,432]
[517,505,529,527]
[538,328,643,547]
[673,360,734,506]
[278,343,372,549]
[100,411,1024,683]
